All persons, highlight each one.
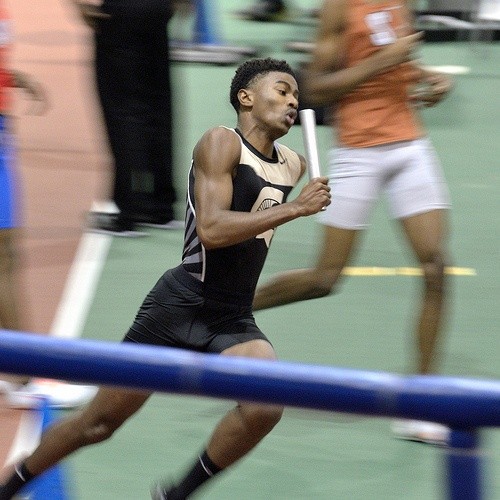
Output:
[1,58,332,500]
[1,1,89,410]
[82,0,177,231]
[251,1,452,446]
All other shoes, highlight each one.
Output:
[389,418,451,444]
